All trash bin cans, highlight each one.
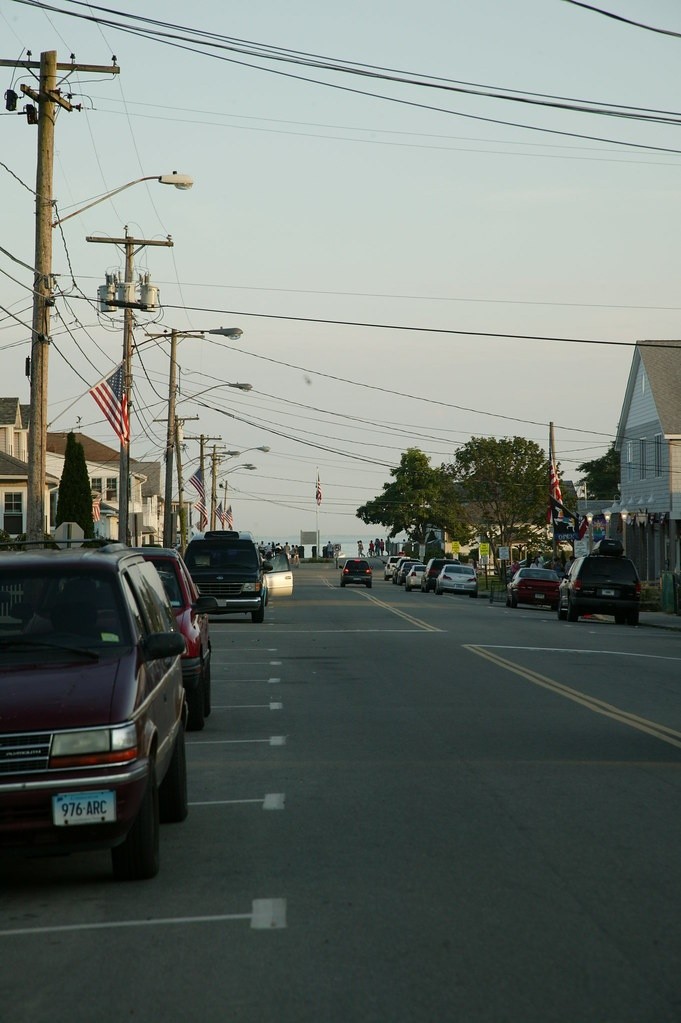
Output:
[299,546,304,558]
[662,570,681,615]
[312,546,316,557]
[323,546,327,557]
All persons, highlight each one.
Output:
[510,555,577,577]
[357,536,391,558]
[327,541,333,558]
[258,541,299,565]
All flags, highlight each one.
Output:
[197,515,208,529]
[189,468,206,501]
[89,366,130,447]
[214,505,224,524]
[317,474,322,505]
[195,497,209,522]
[223,508,233,530]
[549,496,581,541]
[547,457,564,524]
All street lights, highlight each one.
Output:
[162,326,252,548]
[199,434,270,531]
[27,171,194,549]
[153,415,240,551]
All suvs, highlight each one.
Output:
[384,556,403,581]
[184,530,293,623]
[421,558,461,593]
[558,537,641,629]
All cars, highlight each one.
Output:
[506,568,561,610]
[392,557,423,586]
[133,548,212,731]
[406,565,427,591]
[435,564,478,598]
[339,559,373,588]
[0,549,187,881]
